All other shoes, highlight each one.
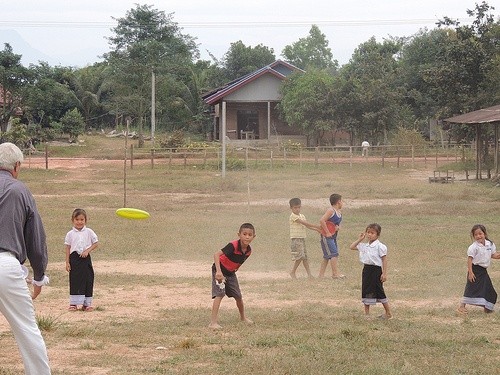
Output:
[456,308,468,315]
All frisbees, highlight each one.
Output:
[116,208,151,220]
[319,221,336,237]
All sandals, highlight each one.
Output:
[82,305,93,312]
[68,305,77,311]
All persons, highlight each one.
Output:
[64,208,99,312]
[320,194,346,280]
[208,223,256,328]
[289,198,323,279]
[456,224,500,314]
[0,142,51,375]
[362,138,370,157]
[350,223,392,319]
